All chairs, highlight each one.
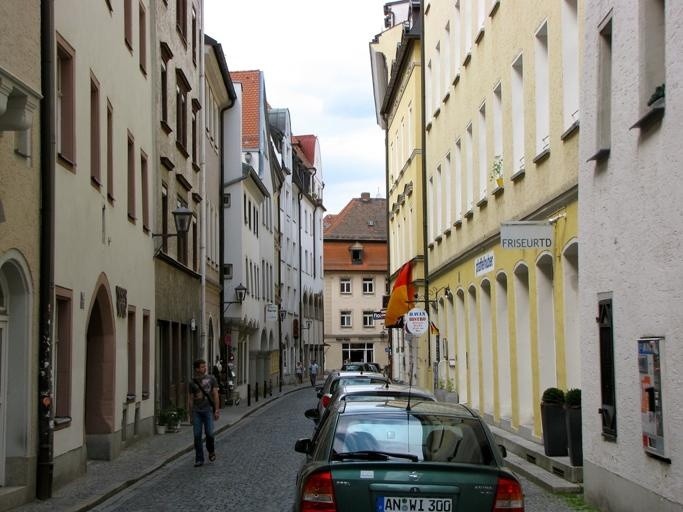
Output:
[427,430,462,461]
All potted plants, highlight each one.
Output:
[487,158,503,186]
[563,388,582,466]
[156,406,187,434]
[540,387,568,457]
[445,377,459,403]
[435,379,445,401]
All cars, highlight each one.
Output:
[294,362,525,512]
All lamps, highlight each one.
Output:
[223,282,247,314]
[301,317,313,329]
[278,306,287,322]
[152,207,194,260]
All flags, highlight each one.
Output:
[383,262,439,336]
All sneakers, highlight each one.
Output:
[194,461,202,467]
[209,452,215,461]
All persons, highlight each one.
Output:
[308,360,318,383]
[296,361,305,383]
[310,360,318,386]
[188,359,220,467]
